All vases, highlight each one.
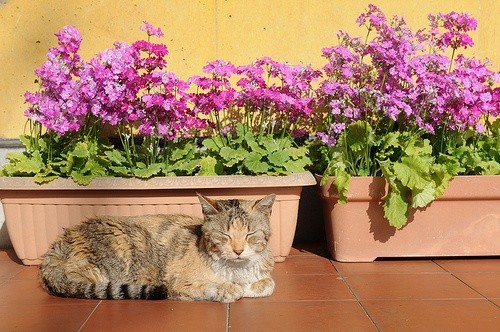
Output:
[315,173,500,263]
[0,170,317,265]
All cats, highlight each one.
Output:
[36,192,278,303]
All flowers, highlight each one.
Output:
[310,0,499,229]
[0,20,322,186]
